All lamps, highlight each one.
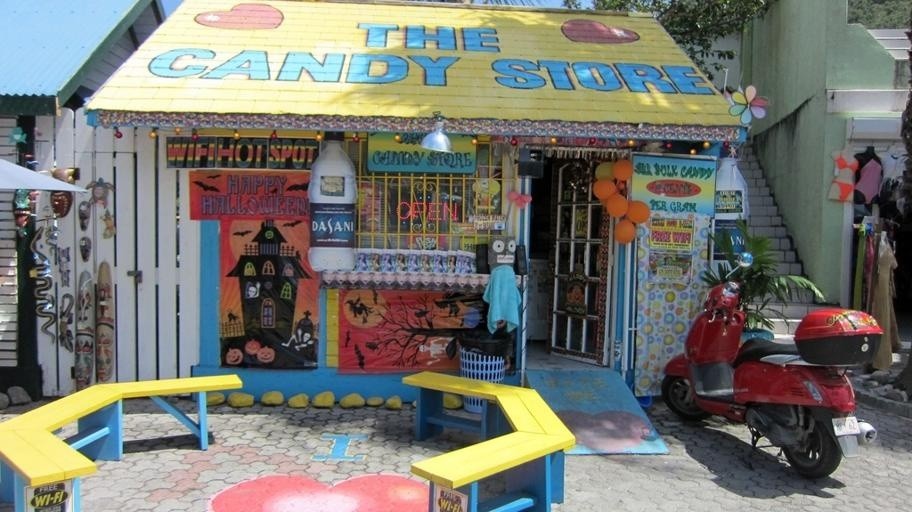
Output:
[421,119,453,155]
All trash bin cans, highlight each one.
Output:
[454,329,510,414]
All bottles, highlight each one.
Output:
[716,148,749,260]
[307,135,357,272]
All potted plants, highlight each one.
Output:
[701,213,826,342]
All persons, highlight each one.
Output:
[852,146,884,206]
[877,145,907,196]
[826,143,860,204]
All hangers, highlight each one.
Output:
[872,218,900,236]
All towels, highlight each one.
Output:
[483,265,522,335]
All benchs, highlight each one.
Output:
[402,372,578,512]
[0,375,244,512]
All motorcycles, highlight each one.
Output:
[660,249,882,477]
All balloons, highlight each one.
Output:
[592,158,651,245]
